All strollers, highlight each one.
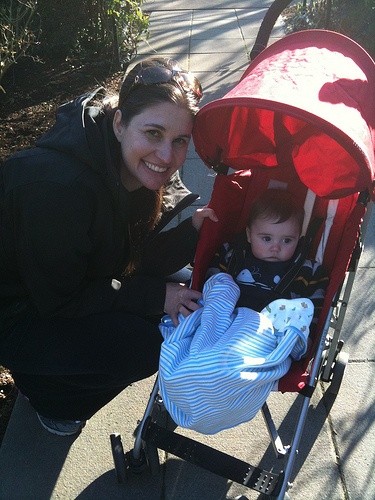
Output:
[110,1,375,500]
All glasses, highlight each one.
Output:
[119,67,204,106]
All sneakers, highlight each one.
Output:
[37,411,85,437]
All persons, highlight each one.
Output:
[210,188,330,361]
[1,58,219,437]
